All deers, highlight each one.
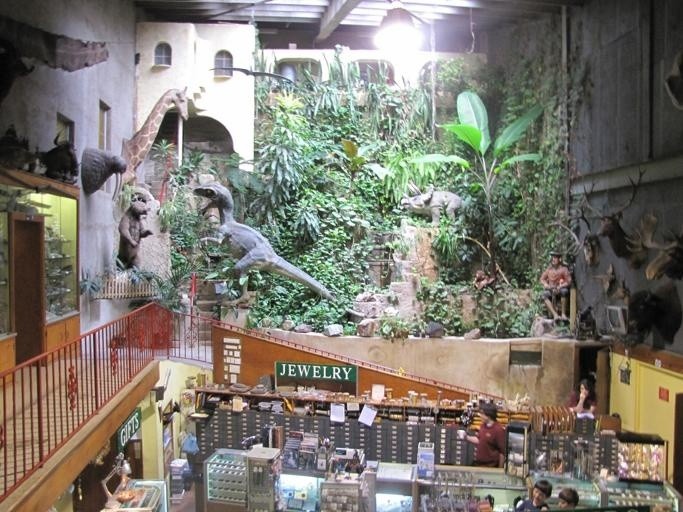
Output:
[622,207,682,282]
[548,211,601,268]
[578,165,647,258]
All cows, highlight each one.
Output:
[619,283,683,345]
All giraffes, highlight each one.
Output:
[121,86,191,195]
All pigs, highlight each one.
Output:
[399,182,465,228]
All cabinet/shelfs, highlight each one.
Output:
[101,381,683,511]
[0,170,79,386]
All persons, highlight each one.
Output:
[539,251,573,322]
[557,487,580,509]
[511,479,551,512]
[456,401,505,469]
[566,376,598,415]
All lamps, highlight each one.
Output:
[605,305,630,337]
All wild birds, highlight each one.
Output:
[210,65,300,88]
